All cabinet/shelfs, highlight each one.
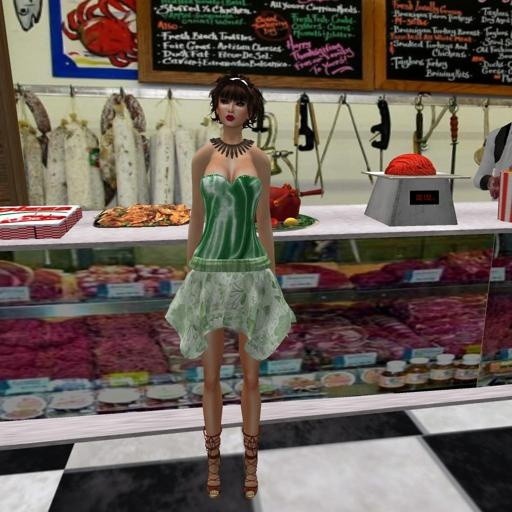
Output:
[0,200,512,450]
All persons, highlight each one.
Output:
[474,121,512,199]
[164,73,297,499]
